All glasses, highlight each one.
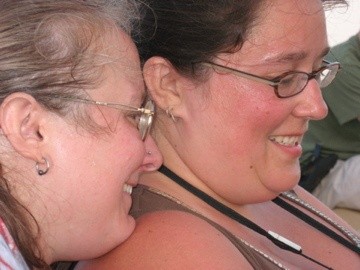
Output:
[201,60,342,98]
[32,93,157,142]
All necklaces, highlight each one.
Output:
[135,192,360,270]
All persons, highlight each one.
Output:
[298,32,360,211]
[0,0,163,270]
[54,1,360,270]
